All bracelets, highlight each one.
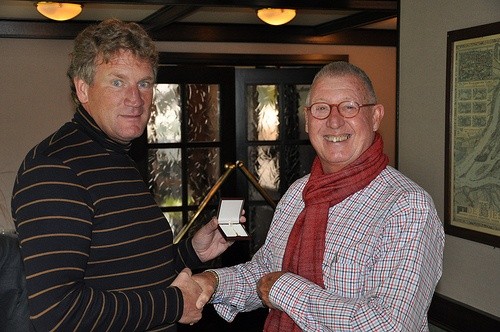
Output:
[204,270,219,287]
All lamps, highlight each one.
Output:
[257,8,296,25]
[35,2,82,22]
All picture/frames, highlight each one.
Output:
[440,20,499,249]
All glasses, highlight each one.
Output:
[307,100,376,119]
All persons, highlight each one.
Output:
[191,60,446,332]
[11,18,245,332]
